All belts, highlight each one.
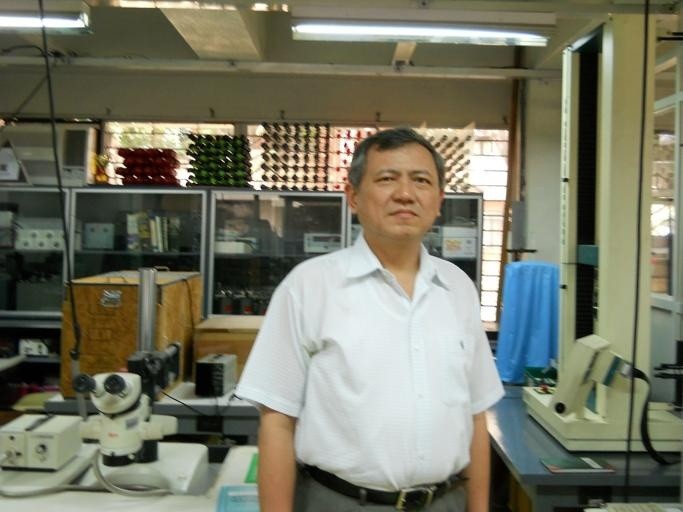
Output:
[297,463,469,512]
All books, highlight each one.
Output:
[126,213,181,253]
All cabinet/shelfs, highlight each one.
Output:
[1,183,483,380]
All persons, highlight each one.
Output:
[233,128,506,512]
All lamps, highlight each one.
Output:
[0,11,89,28]
[288,18,548,50]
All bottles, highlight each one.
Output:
[114,118,475,196]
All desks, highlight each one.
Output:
[468,383,683,512]
